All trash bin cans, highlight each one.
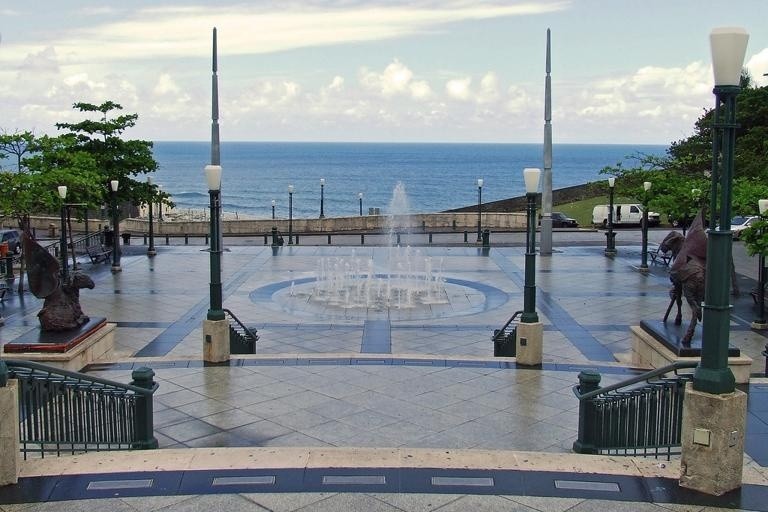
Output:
[48,223,58,237]
[122,233,130,245]
[482,228,490,247]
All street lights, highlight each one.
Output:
[679,27,749,496]
[640,181,652,270]
[604,177,618,255]
[515,167,544,366]
[271,179,364,246]
[202,164,231,367]
[57,176,163,286]
[750,199,768,328]
[477,179,483,242]
[692,188,701,212]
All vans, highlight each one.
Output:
[0,230,21,254]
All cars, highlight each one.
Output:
[715,216,768,240]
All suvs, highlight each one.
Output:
[538,212,578,227]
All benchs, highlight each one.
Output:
[84,242,112,267]
[647,245,674,270]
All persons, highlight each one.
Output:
[100,225,113,246]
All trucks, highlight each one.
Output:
[592,203,661,228]
